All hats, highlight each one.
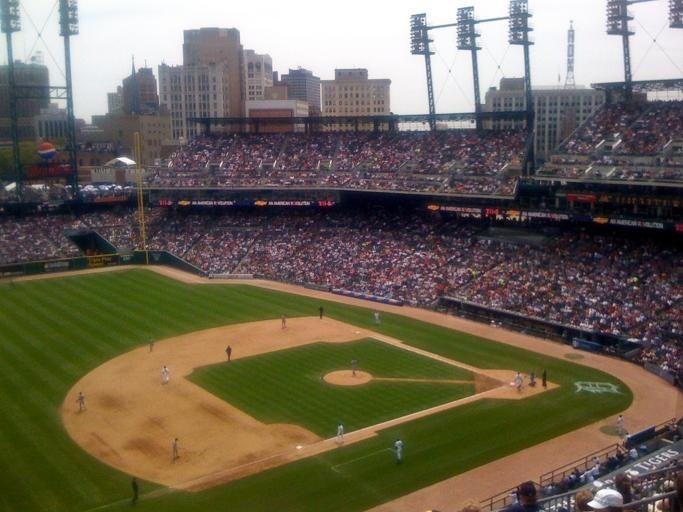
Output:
[587,490,624,509]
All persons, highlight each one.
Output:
[2,106,682,512]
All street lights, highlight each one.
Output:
[408,13,437,131]
[0,0,23,190]
[605,0,635,99]
[56,0,80,200]
[506,0,534,130]
[665,0,682,30]
[454,7,483,128]
